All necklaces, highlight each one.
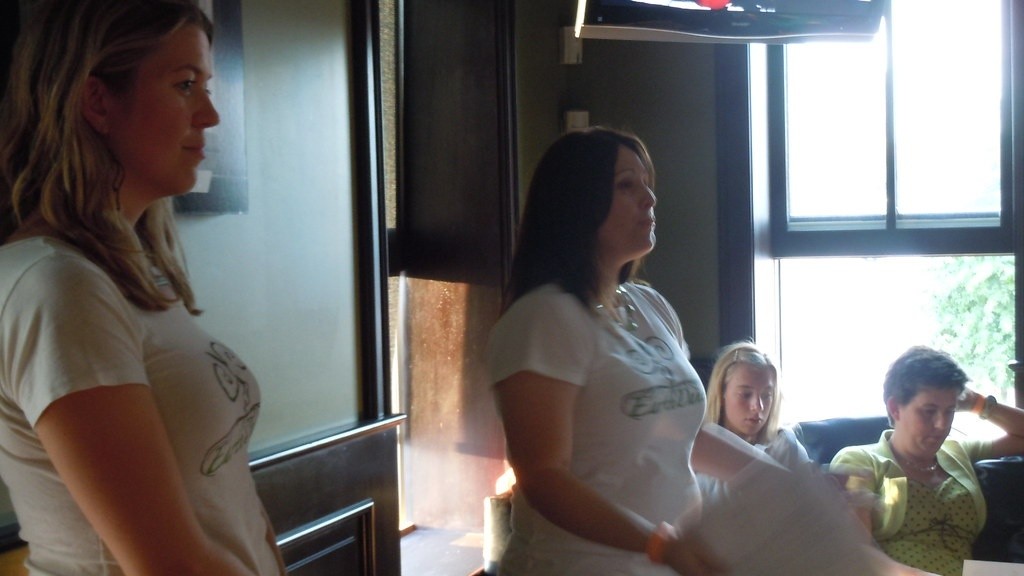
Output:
[898,456,938,470]
[595,285,639,331]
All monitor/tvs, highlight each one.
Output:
[572,0,886,45]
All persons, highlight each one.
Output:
[482,128,1024,576]
[0,0,286,576]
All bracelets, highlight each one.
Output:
[980,395,996,419]
[647,520,672,564]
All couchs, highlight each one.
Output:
[776,416,1024,563]
